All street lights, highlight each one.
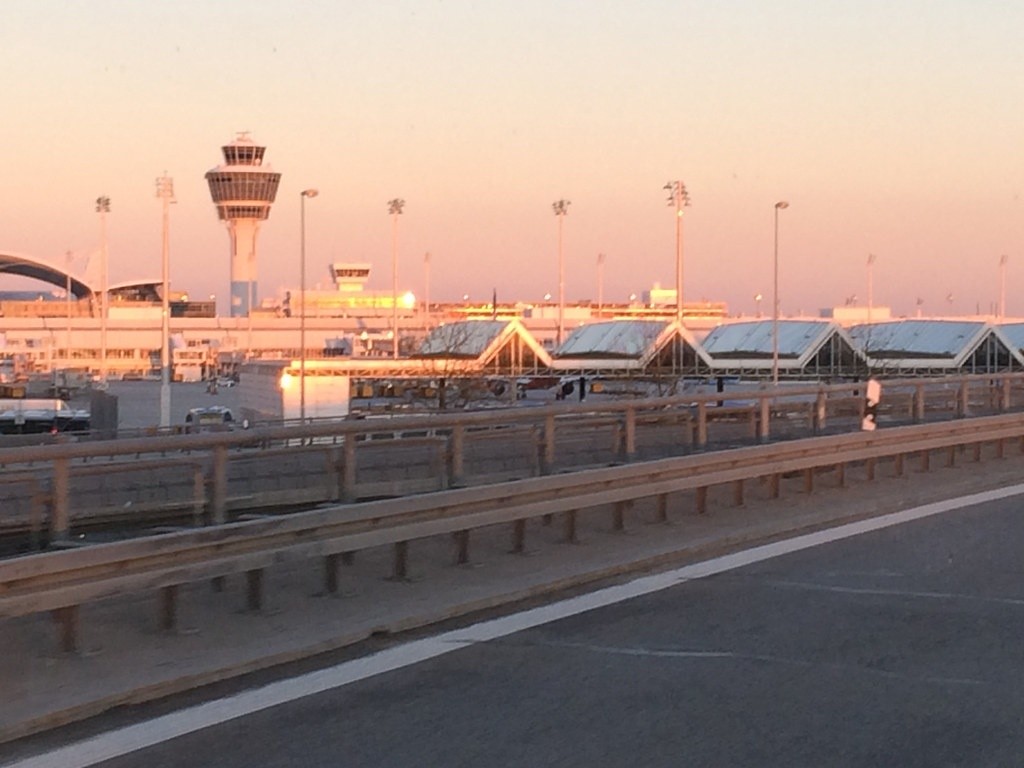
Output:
[773,201,790,389]
[662,178,692,377]
[154,169,176,432]
[386,197,407,361]
[423,251,432,334]
[999,253,1010,321]
[94,195,112,387]
[299,189,319,425]
[552,198,573,350]
[867,253,877,379]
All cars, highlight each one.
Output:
[185,406,236,435]
